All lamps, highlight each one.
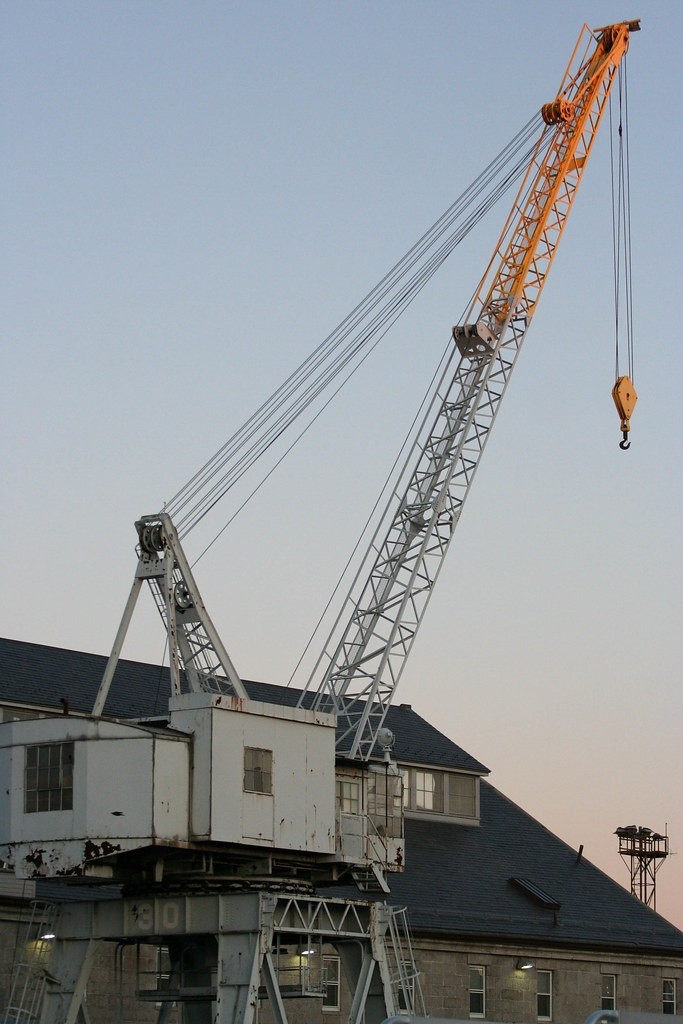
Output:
[298,945,317,955]
[517,958,536,970]
[40,926,55,939]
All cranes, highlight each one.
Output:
[0,18,644,1024]
